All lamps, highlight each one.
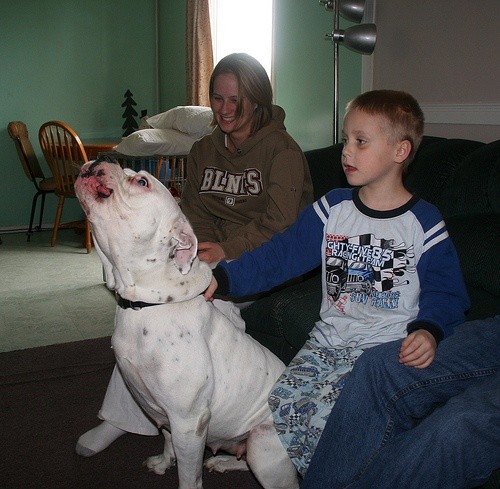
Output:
[326,23,378,55]
[319,0,365,24]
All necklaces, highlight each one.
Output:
[229,136,239,149]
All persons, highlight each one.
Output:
[205,87,470,480]
[70,53,315,455]
[303,311,500,489]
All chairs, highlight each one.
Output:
[7,119,78,242]
[38,121,95,254]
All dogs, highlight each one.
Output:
[74,155,299,489]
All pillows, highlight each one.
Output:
[145,106,214,135]
[112,129,197,156]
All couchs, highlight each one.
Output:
[241,134,500,370]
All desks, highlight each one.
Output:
[48,139,119,159]
